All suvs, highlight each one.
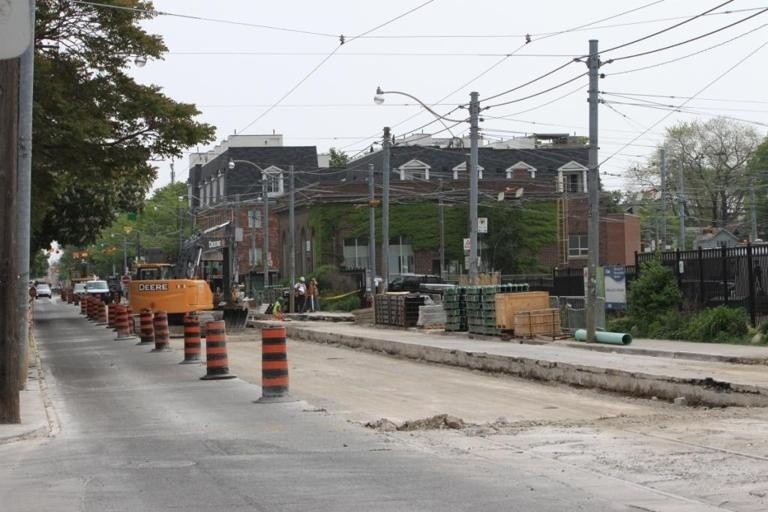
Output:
[33,283,52,300]
[370,272,444,293]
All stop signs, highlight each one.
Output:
[121,275,130,285]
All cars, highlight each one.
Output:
[73,283,85,295]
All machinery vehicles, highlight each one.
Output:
[127,244,216,326]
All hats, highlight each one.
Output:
[299,276,305,282]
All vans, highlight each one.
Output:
[83,280,108,298]
[566,247,588,261]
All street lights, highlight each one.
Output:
[110,231,128,275]
[372,85,483,284]
[176,192,216,240]
[228,156,270,286]
[149,205,184,253]
[250,195,262,273]
[99,242,117,278]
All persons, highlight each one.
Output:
[273,276,320,321]
[29,284,36,303]
[100,273,130,306]
